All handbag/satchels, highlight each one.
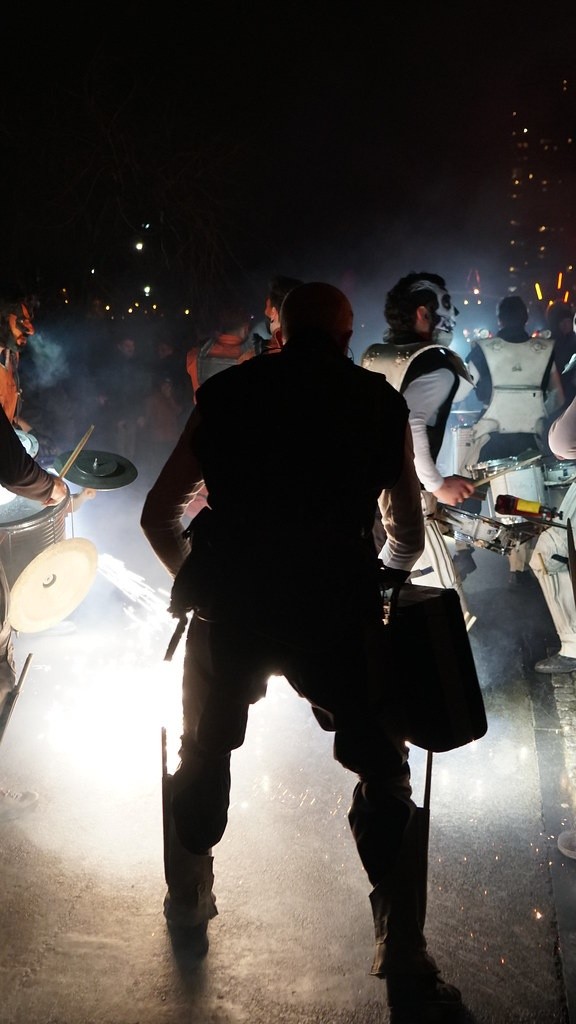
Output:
[372,582,489,753]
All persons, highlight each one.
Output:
[1,274,576,739]
[139,281,463,1024]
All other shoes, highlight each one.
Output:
[534,653,576,674]
[375,955,461,1024]
[163,888,215,952]
[557,831,576,859]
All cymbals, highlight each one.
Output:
[7,537,97,633]
[53,449,139,491]
[74,454,118,476]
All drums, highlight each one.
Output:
[436,423,475,477]
[14,429,40,462]
[0,481,70,592]
[540,460,576,490]
[432,501,517,556]
[463,454,549,526]
